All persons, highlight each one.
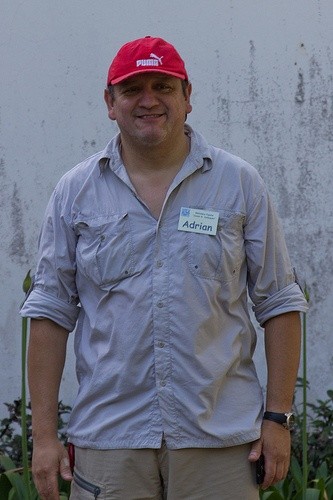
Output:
[17,35,310,500]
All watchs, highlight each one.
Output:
[263,411,296,430]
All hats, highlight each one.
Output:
[107,35,189,81]
